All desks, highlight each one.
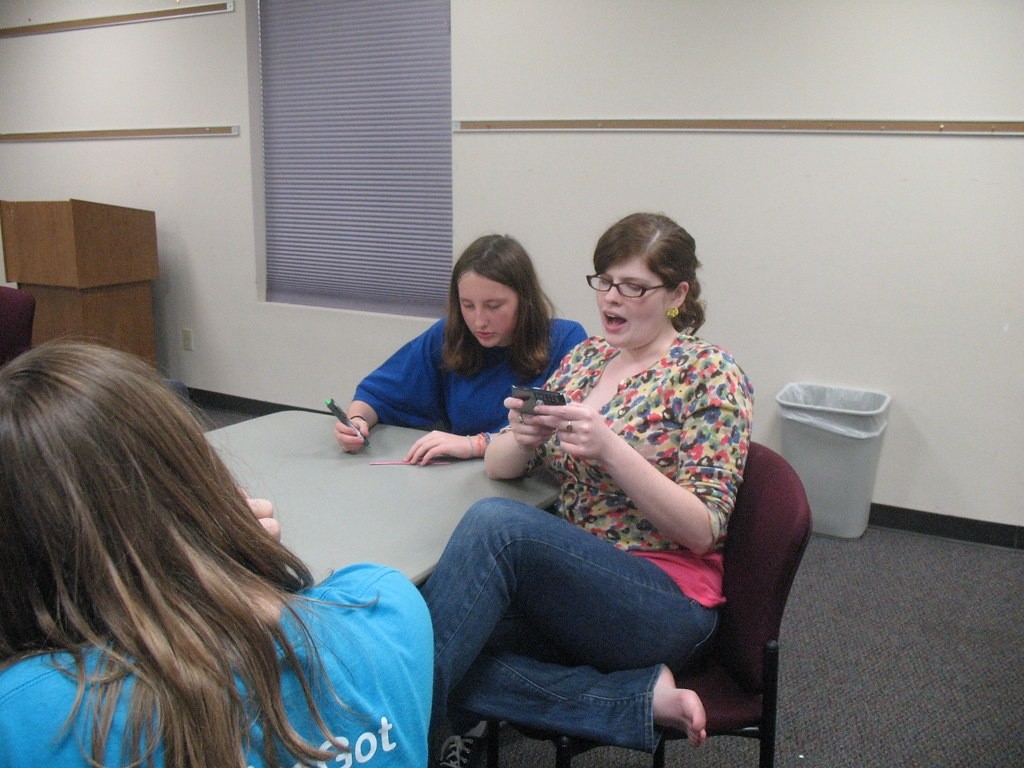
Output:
[203,411,563,589]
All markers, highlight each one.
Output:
[326,398,372,449]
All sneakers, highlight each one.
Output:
[440,719,489,768]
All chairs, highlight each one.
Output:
[0,285,35,370]
[486,440,813,768]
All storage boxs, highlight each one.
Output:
[17,282,156,369]
[0,198,159,289]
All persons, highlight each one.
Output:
[334,232,589,466]
[0,333,435,768]
[419,211,756,756]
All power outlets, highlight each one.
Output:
[182,328,193,350]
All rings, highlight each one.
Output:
[566,420,573,434]
[518,412,524,424]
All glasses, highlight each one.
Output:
[587,274,668,298]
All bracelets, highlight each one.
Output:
[349,415,368,423]
[467,431,493,458]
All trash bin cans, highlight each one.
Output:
[775,381,892,540]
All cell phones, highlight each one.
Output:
[512,385,566,416]
